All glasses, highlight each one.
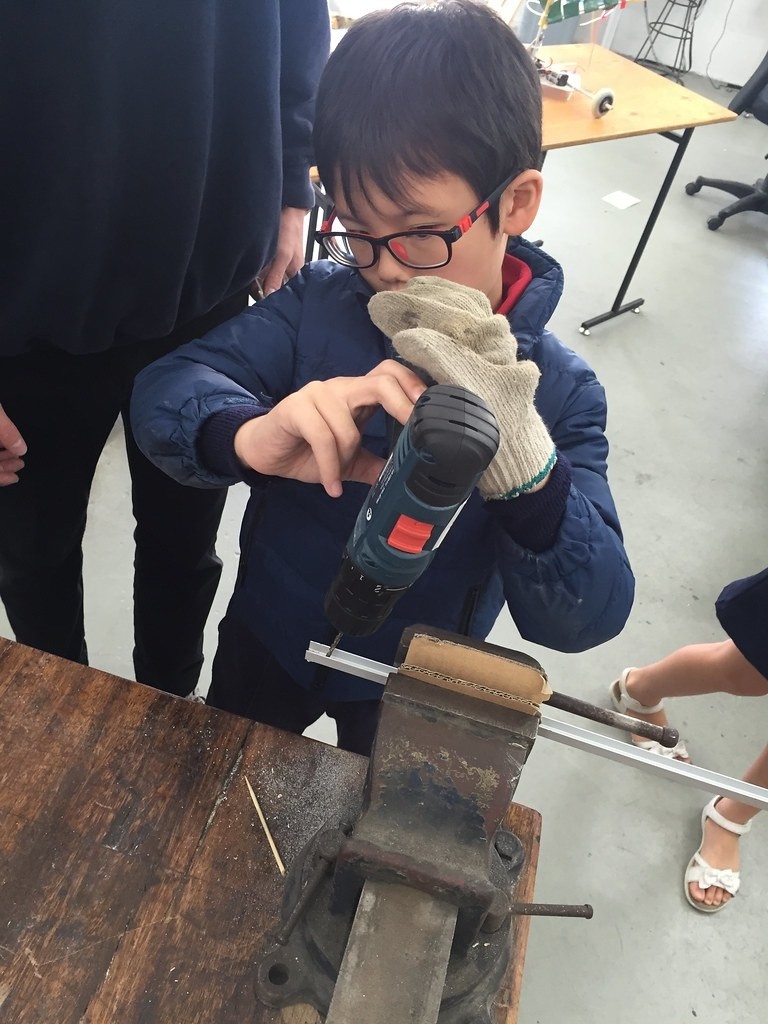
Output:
[313,163,523,269]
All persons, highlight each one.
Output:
[0,1,332,697]
[607,568,768,914]
[129,1,634,757]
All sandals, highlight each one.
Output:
[609,667,691,764]
[683,795,753,914]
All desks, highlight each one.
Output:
[303,42,740,340]
[0,635,542,1024]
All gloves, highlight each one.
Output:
[367,275,559,500]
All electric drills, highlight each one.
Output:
[321,384,502,660]
